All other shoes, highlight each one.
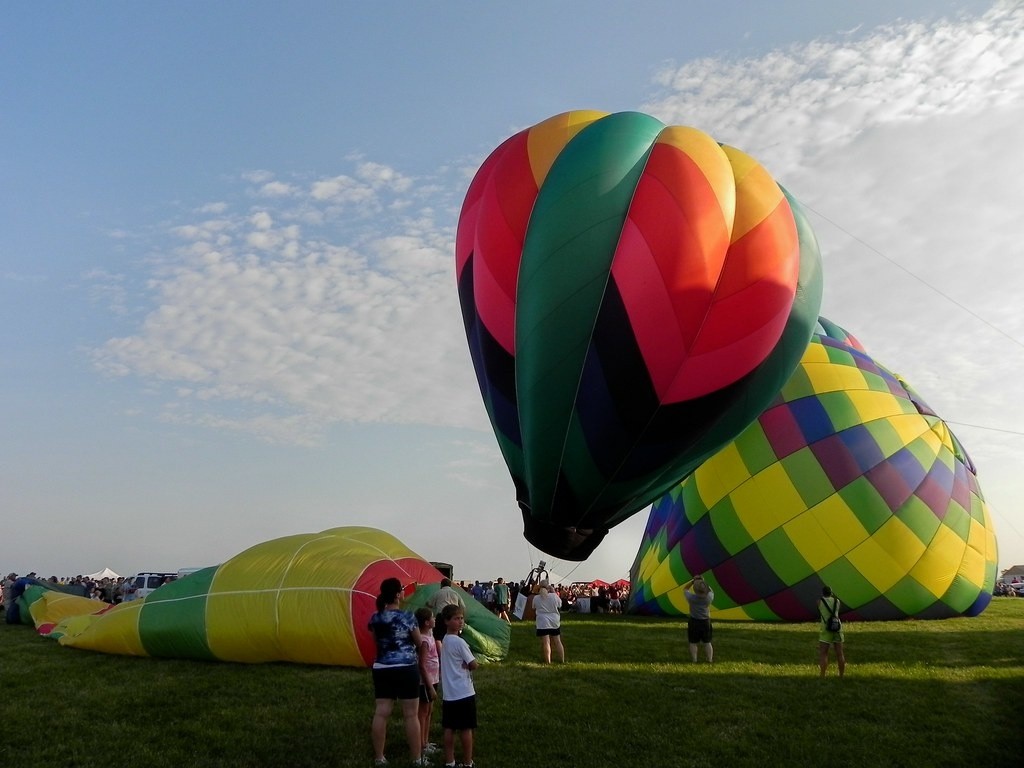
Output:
[462,763,475,768]
[412,755,434,767]
[446,763,463,768]
[423,743,442,755]
[375,756,391,766]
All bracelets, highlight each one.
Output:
[691,580,693,584]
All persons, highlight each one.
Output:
[368,577,435,768]
[458,580,630,615]
[427,578,466,642]
[1012,577,1018,583]
[683,575,713,662]
[816,586,845,678]
[0,572,137,625]
[161,577,170,586]
[532,580,565,664]
[413,608,441,755]
[494,578,511,625]
[440,604,478,768]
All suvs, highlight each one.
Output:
[122,571,178,601]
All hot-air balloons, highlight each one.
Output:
[574,316,999,623]
[6,526,511,668]
[455,108,823,622]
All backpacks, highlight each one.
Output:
[819,597,841,632]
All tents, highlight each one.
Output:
[587,579,631,589]
[82,568,126,581]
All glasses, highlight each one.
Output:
[400,586,405,590]
